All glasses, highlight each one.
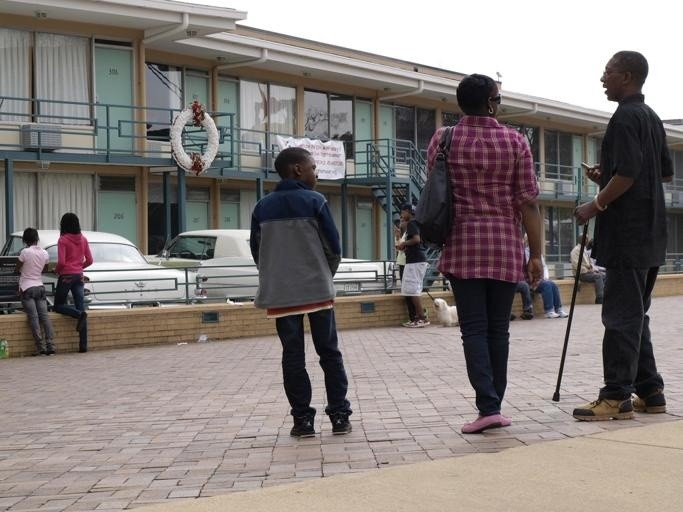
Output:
[493,94,501,103]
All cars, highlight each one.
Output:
[1,229,209,311]
[144,230,395,305]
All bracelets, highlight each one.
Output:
[594,195,608,212]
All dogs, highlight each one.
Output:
[433,298,459,327]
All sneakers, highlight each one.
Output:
[574,399,634,421]
[557,310,568,318]
[502,415,512,425]
[401,320,412,326]
[631,391,666,413]
[544,312,559,317]
[331,414,352,435]
[291,422,314,438]
[461,415,501,433]
[409,320,430,327]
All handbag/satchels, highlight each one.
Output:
[416,126,453,243]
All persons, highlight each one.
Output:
[397,203,431,328]
[509,281,534,321]
[18,227,57,357]
[573,52,674,420]
[585,238,609,293]
[415,74,545,432]
[249,148,353,438]
[52,212,93,353]
[523,232,569,318]
[391,215,418,328]
[570,234,606,304]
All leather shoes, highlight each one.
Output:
[77,312,87,332]
